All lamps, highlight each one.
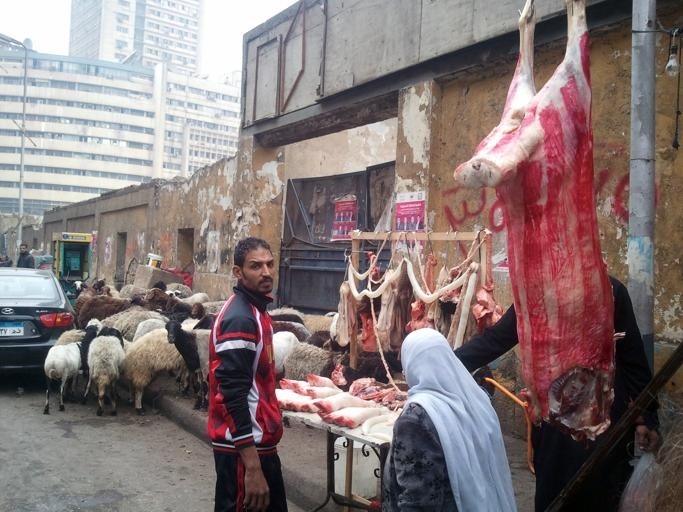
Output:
[662,23,682,78]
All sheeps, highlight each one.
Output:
[42,277,494,417]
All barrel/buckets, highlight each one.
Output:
[333,436,380,499]
[146,252,164,267]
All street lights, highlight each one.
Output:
[0,32,29,265]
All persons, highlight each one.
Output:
[452,278,663,510]
[208,236,288,510]
[0,251,13,267]
[17,244,35,268]
[389,327,518,512]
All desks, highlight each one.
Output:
[279,407,394,512]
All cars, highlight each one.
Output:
[0,263,79,384]
